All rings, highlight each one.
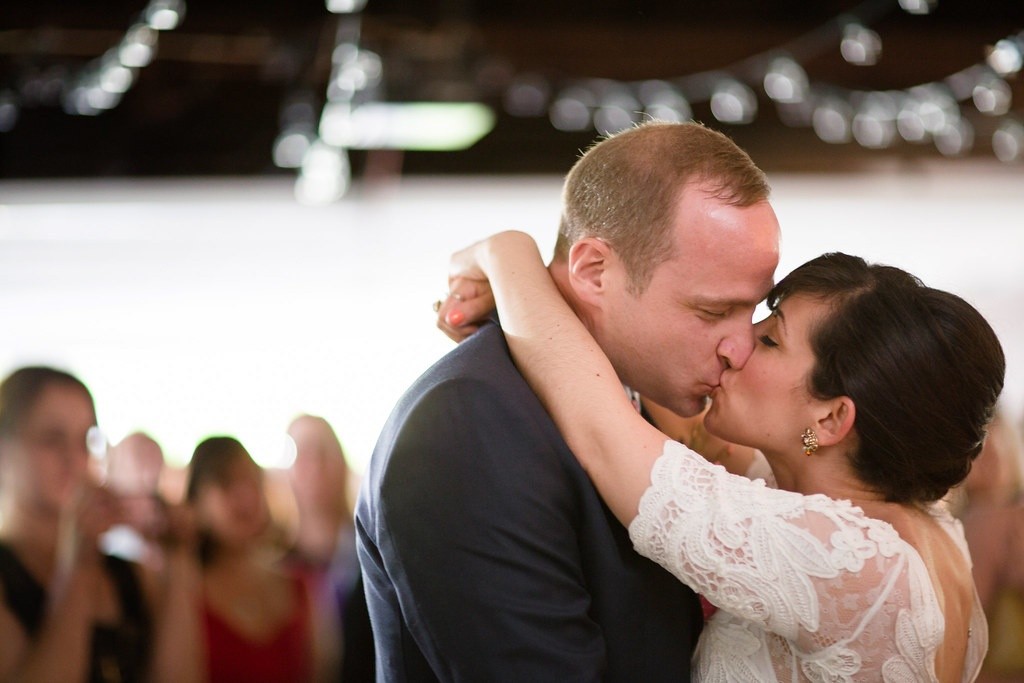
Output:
[433,299,443,313]
[449,292,465,301]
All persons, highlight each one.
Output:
[0,366,364,683]
[943,411,1024,682]
[354,121,782,682]
[438,229,1005,683]
